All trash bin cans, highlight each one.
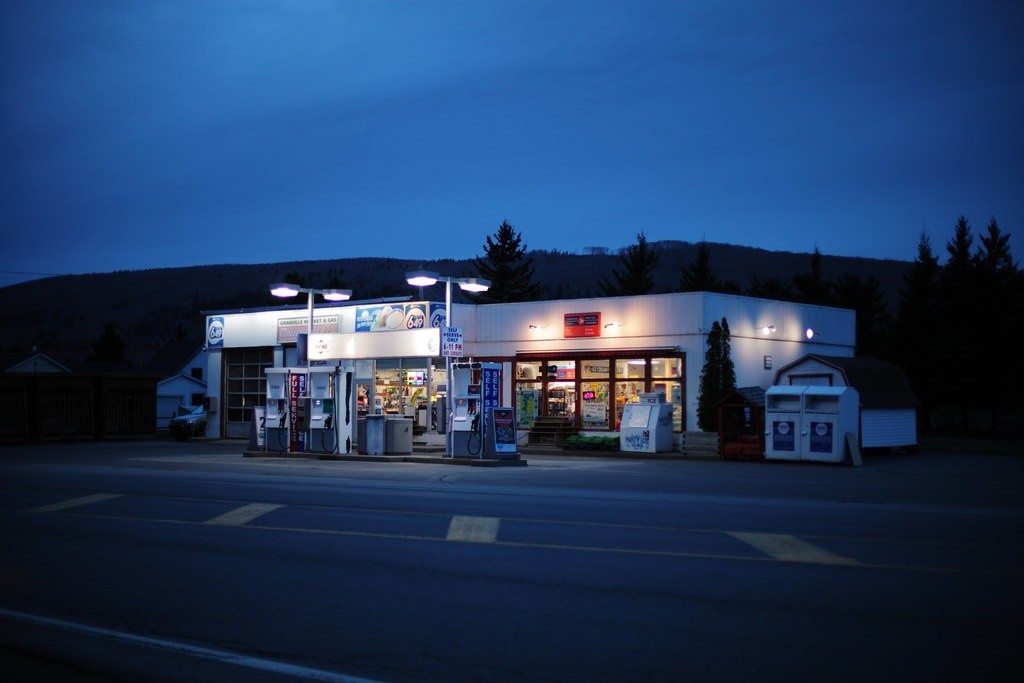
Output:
[366,414,387,456]
[619,392,674,453]
[765,384,861,463]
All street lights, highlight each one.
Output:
[269,281,352,452]
[406,270,491,458]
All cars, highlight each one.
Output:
[170,403,207,440]
